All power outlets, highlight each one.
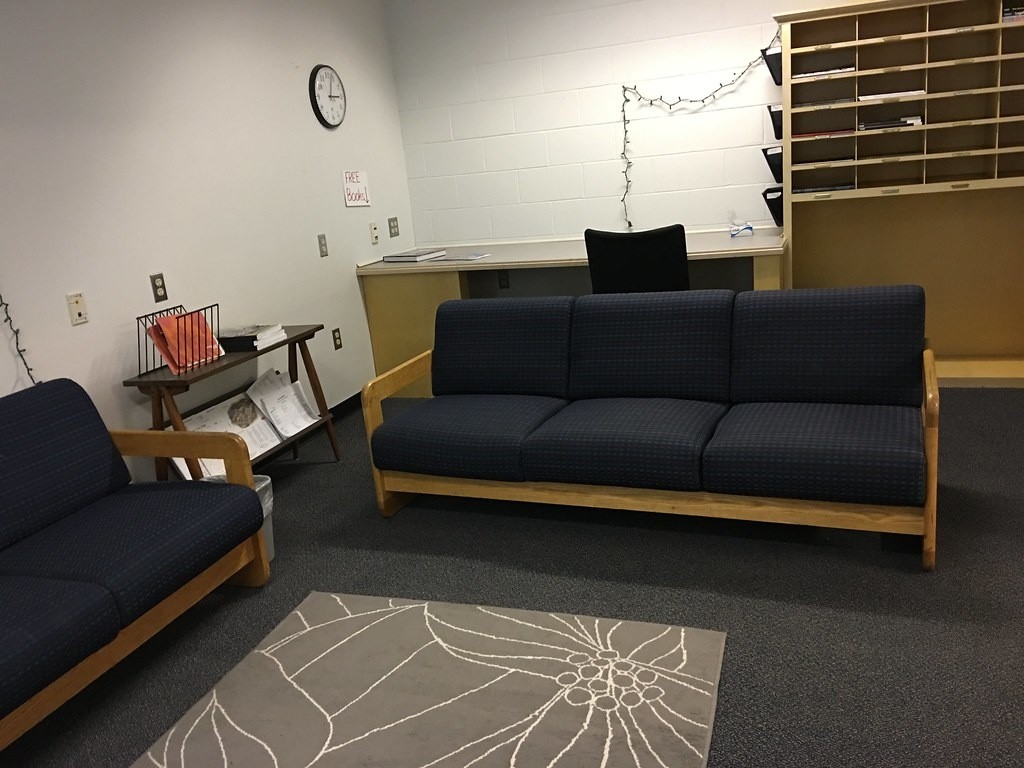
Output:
[332,327,343,351]
[150,273,168,304]
[318,234,329,257]
[388,217,399,239]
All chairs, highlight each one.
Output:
[584,223,690,294]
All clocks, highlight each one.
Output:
[309,64,346,129]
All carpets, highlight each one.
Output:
[129,590,726,768]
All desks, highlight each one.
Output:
[122,324,342,480]
[355,227,785,375]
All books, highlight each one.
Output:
[383,248,446,262]
[1003,7,1024,17]
[214,323,287,351]
[859,116,922,130]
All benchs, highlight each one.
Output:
[360,283,942,569]
[0,378,274,752]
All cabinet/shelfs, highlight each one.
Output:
[772,0,1024,388]
